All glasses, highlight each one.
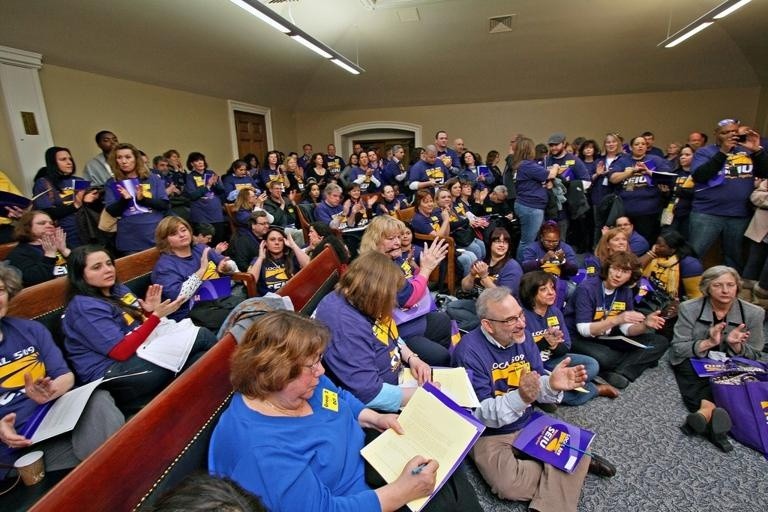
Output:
[296,351,326,370]
[480,307,526,326]
[493,239,512,246]
[617,223,632,228]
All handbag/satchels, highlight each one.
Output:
[432,293,459,313]
[456,286,484,300]
[450,226,475,247]
[710,366,768,458]
[598,193,626,227]
[632,277,672,316]
[187,295,245,330]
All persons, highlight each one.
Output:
[207,308,485,512]
[667,264,766,454]
[153,470,268,512]
[3,116,767,413]
[449,284,617,511]
[1,258,126,473]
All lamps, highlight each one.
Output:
[655,0,752,52]
[229,0,367,76]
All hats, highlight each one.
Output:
[547,133,567,144]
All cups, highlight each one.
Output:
[12,450,45,487]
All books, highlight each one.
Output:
[29,367,151,443]
[510,410,598,476]
[359,379,486,511]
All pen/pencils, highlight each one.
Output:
[411,463,428,475]
[732,359,750,367]
[565,444,594,459]
[429,368,434,382]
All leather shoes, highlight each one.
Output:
[592,379,619,398]
[602,370,628,388]
[586,452,616,478]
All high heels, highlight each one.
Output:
[678,411,708,437]
[708,407,734,452]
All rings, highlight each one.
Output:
[8,444,16,448]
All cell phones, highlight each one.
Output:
[263,189,268,198]
[434,177,444,186]
[732,134,748,142]
